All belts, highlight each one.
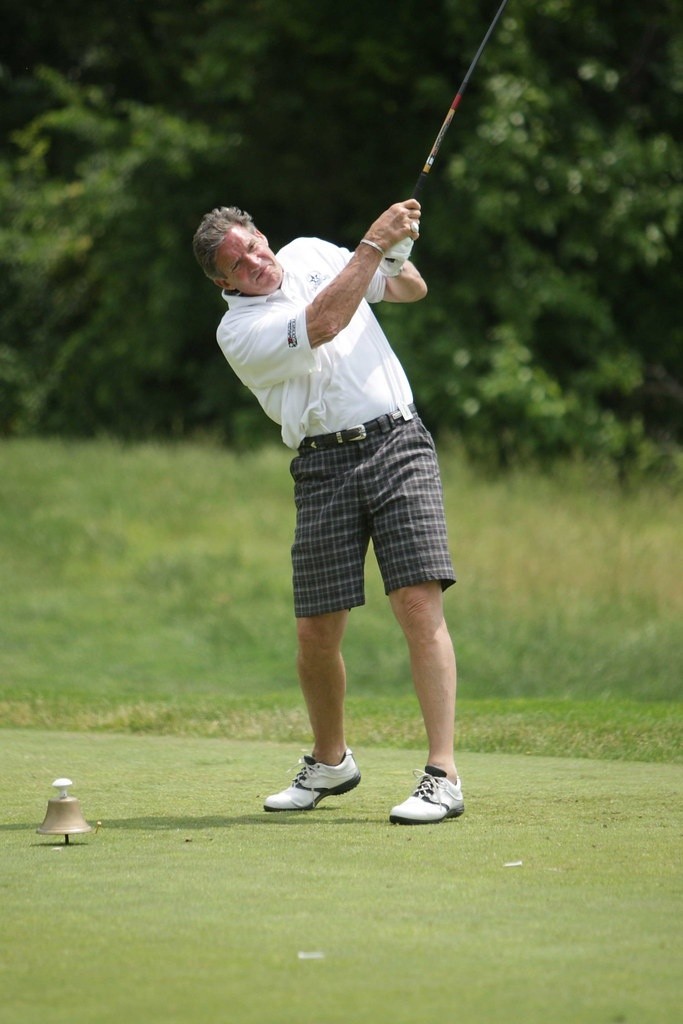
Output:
[299,403,417,450]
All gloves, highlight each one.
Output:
[378,221,419,276]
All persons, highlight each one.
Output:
[193,199,465,825]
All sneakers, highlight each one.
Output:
[389,764,465,825]
[262,748,361,812]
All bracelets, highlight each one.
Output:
[360,239,384,255]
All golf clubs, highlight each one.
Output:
[384,1,506,264]
